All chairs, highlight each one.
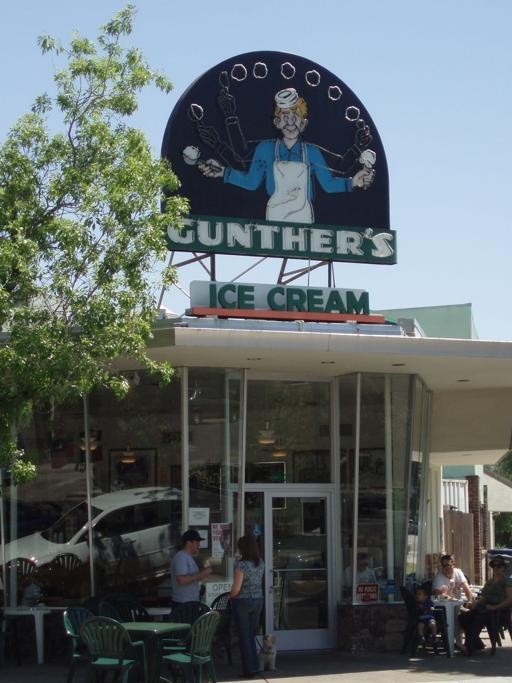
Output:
[2,552,263,682]
[398,574,511,662]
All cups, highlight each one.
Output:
[388,594,394,601]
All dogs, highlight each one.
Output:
[257,633,278,672]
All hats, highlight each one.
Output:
[182,529,205,541]
[489,556,509,565]
[441,555,454,563]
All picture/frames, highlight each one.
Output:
[232,459,287,510]
[290,447,393,537]
[168,460,222,515]
[106,447,161,493]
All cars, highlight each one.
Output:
[6,464,100,518]
[0,486,184,593]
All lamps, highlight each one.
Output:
[120,442,137,464]
[79,414,98,453]
[255,382,278,445]
[271,435,290,458]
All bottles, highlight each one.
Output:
[429,587,467,601]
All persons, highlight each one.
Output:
[413,587,438,648]
[198,88,377,225]
[343,552,376,592]
[432,554,473,651]
[169,530,212,639]
[227,536,266,679]
[465,554,511,652]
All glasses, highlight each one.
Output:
[491,564,502,568]
